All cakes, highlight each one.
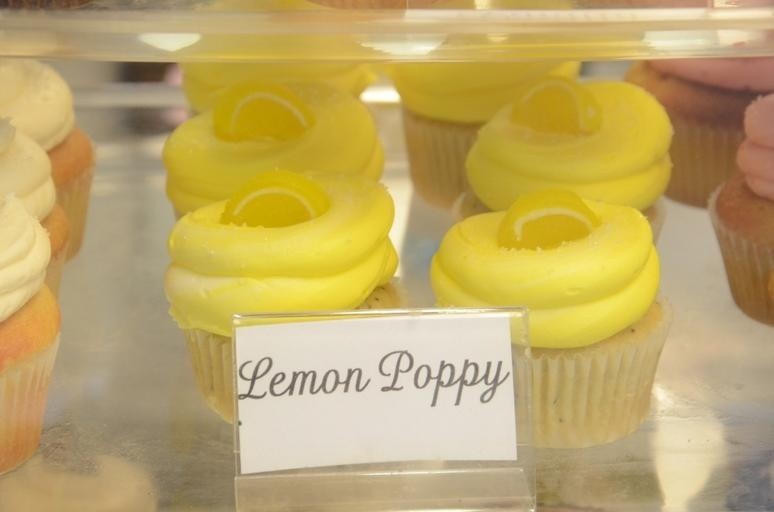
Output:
[181,63,369,113]
[430,186,674,450]
[390,62,581,206]
[0,189,60,479]
[625,57,774,211]
[464,72,676,251]
[165,82,388,206]
[713,88,774,325]
[163,172,406,429]
[1,56,95,261]
[2,115,69,265]
